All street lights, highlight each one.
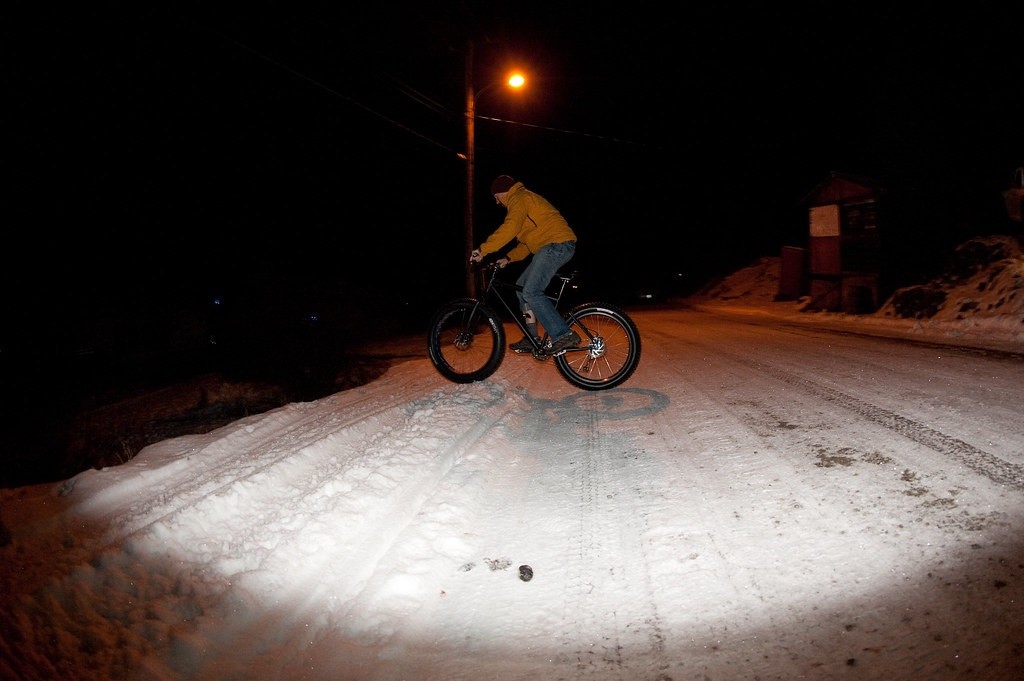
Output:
[466,72,527,294]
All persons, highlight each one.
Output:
[471,176,580,355]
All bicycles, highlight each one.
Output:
[425,252,641,391]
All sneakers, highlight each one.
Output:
[546,331,581,355]
[509,336,542,351]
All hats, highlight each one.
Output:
[491,175,514,195]
